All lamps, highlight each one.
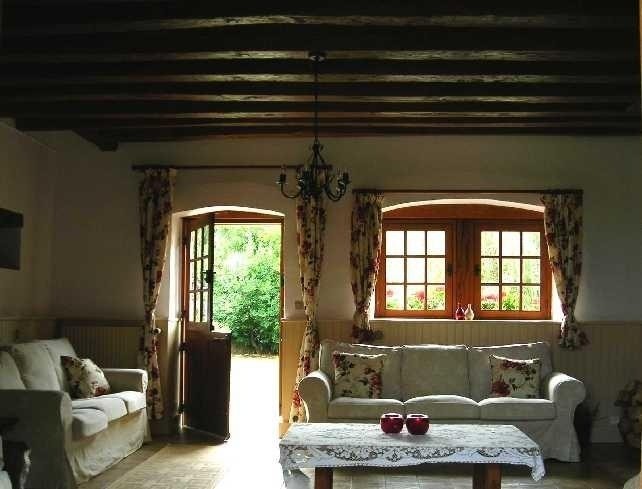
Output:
[276,51,350,201]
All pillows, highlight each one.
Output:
[332,351,388,399]
[487,353,540,399]
[32,337,79,394]
[401,344,471,403]
[60,356,112,398]
[4,343,60,391]
[318,338,401,399]
[0,350,26,390]
[467,341,552,403]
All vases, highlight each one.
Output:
[456,303,465,320]
[464,303,474,320]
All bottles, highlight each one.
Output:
[455,302,474,322]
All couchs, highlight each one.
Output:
[298,337,588,463]
[0,337,152,489]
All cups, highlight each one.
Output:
[378,413,431,436]
